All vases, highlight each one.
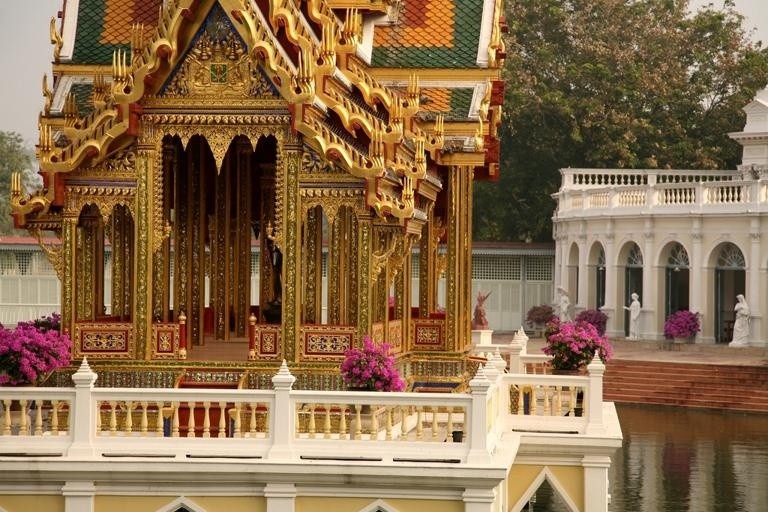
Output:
[348,387,370,414]
[0,382,33,414]
[552,366,587,416]
[674,336,689,344]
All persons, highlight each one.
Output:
[623,289,644,341]
[729,294,752,346]
[554,286,573,322]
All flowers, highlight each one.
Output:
[541,320,611,370]
[340,336,405,392]
[0,312,73,386]
[662,309,700,337]
